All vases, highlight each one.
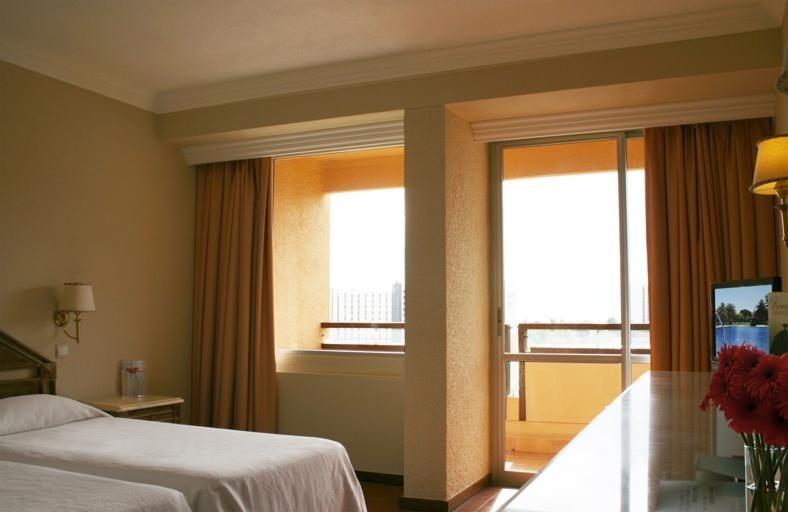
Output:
[745,446,786,512]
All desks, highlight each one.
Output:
[496,367,761,511]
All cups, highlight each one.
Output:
[120,359,145,401]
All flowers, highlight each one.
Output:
[702,344,788,512]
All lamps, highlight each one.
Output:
[56,280,98,344]
[749,134,788,251]
